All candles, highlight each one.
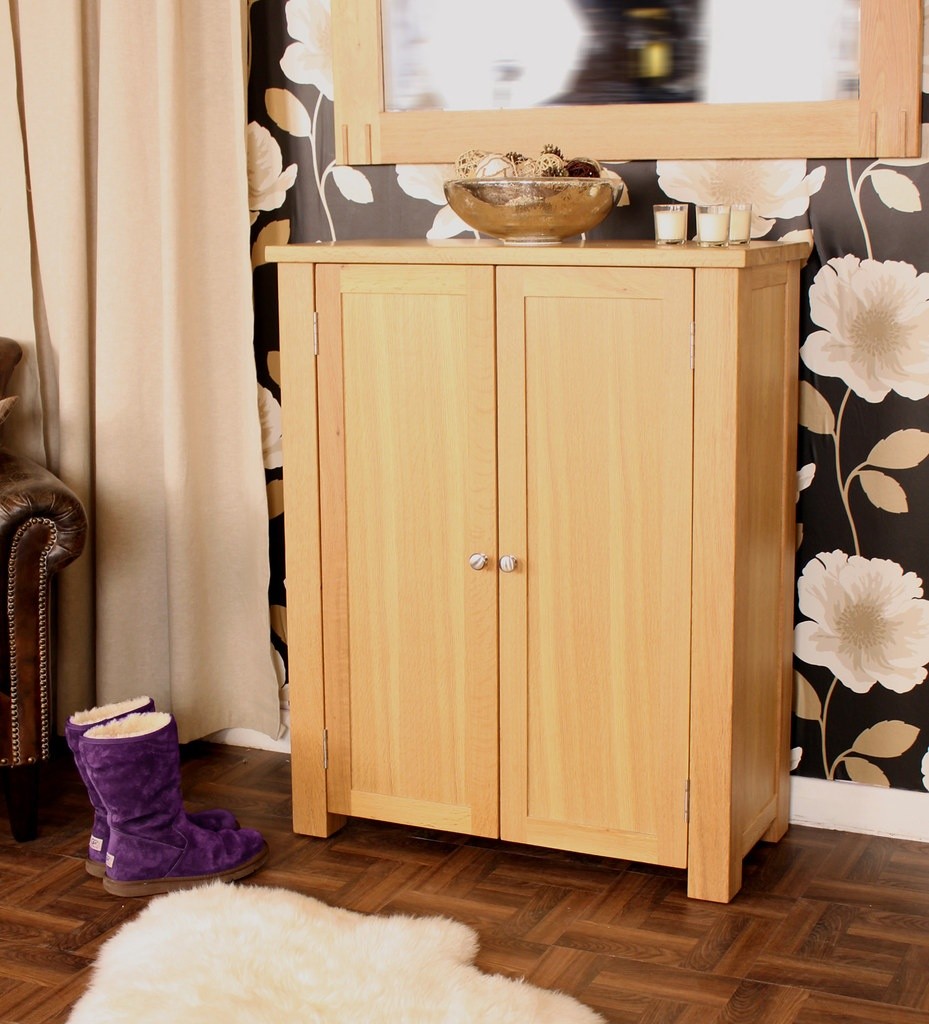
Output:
[728,203,752,246]
[653,204,688,246]
[696,204,730,248]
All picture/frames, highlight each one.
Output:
[331,0,923,166]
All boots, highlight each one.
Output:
[65,694,241,877]
[78,712,273,897]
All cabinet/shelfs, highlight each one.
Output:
[265,238,807,903]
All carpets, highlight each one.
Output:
[66,880,607,1024]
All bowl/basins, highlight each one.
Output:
[442,176,624,247]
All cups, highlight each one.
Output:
[652,203,688,247]
[728,202,753,246]
[695,204,728,249]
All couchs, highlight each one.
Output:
[1,337,89,843]
